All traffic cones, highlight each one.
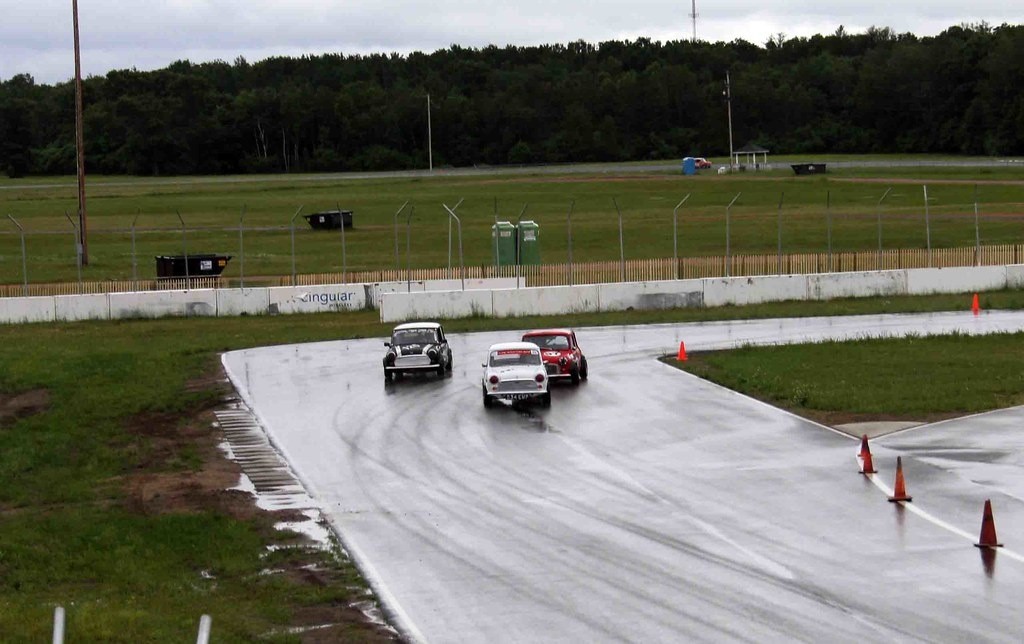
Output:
[971,294,981,310]
[858,447,879,474]
[973,499,1005,547]
[676,340,688,361]
[887,456,912,502]
[857,435,872,457]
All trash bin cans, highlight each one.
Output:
[302,209,352,232]
[154,253,232,278]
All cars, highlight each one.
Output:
[382,322,453,382]
[482,342,551,409]
[693,157,712,169]
[521,327,588,387]
